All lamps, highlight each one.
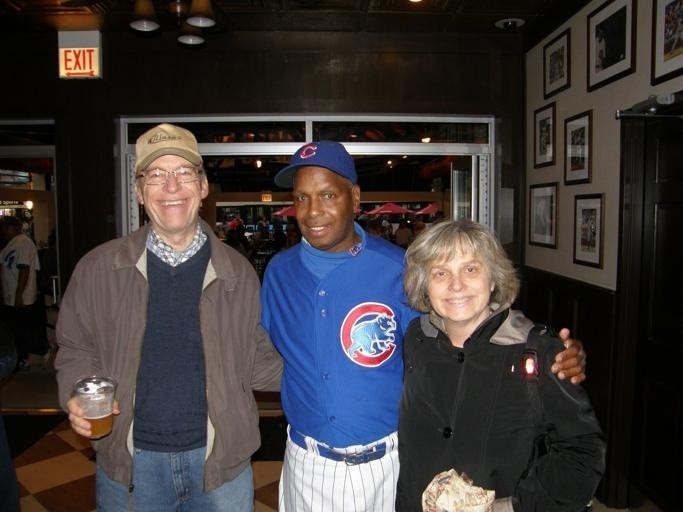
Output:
[130,1,217,46]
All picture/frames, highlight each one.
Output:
[529,181,561,250]
[574,192,606,270]
[650,0,683,86]
[533,101,557,167]
[542,26,572,100]
[587,0,637,92]
[564,108,593,184]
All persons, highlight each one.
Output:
[1,214,51,371]
[394,219,608,512]
[355,210,445,250]
[259,139,589,512]
[53,121,287,512]
[221,210,302,262]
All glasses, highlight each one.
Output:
[138,168,199,186]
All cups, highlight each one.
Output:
[72,374,118,437]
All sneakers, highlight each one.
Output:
[14,359,30,375]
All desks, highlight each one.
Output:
[0,370,285,417]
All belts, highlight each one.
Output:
[290,428,386,466]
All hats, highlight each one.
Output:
[275,140,357,186]
[134,124,202,171]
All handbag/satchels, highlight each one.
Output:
[422,467,496,512]
